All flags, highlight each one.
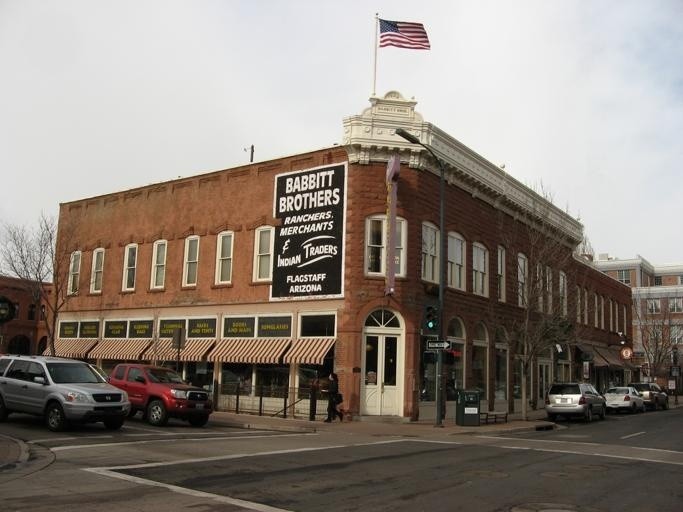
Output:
[377,17,431,51]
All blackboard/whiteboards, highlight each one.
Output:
[58,312,294,338]
[268,161,349,303]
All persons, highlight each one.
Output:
[324,372,345,423]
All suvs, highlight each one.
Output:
[110,364,212,428]
[545,382,607,423]
[0,353,131,433]
[628,382,668,411]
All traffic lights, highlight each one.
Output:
[425,305,439,330]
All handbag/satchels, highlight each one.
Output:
[335,394,343,404]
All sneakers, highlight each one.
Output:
[339,412,342,421]
[324,418,331,422]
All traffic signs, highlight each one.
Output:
[427,340,452,351]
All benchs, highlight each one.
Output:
[480,411,508,424]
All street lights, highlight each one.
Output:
[672,346,678,405]
[396,127,446,429]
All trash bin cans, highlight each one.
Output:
[456,391,480,426]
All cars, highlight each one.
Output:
[604,386,646,414]
